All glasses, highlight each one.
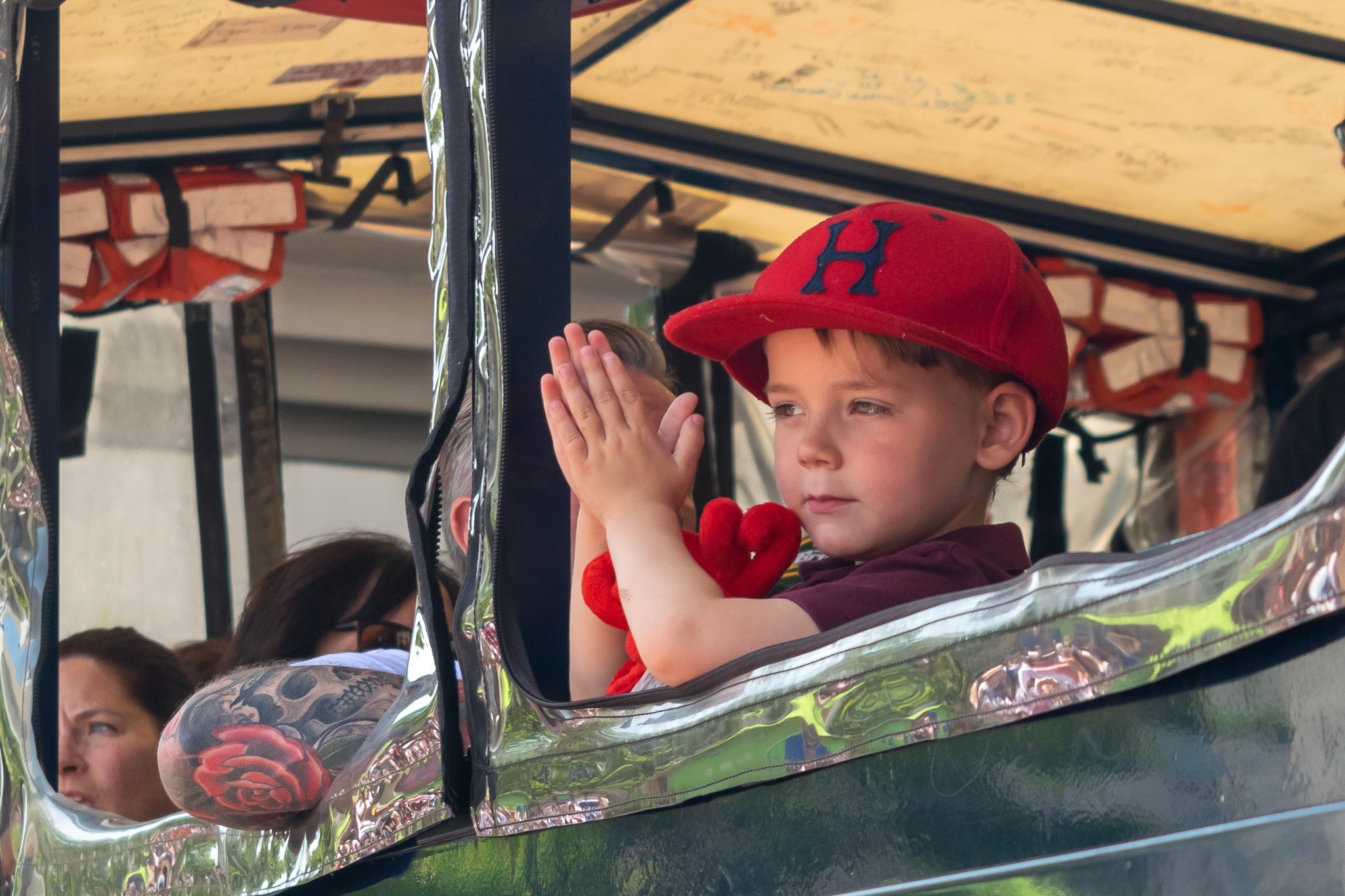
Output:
[324,617,458,663]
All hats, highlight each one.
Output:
[662,202,1068,454]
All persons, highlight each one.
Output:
[56,540,457,824]
[155,318,697,830]
[539,198,1071,711]
